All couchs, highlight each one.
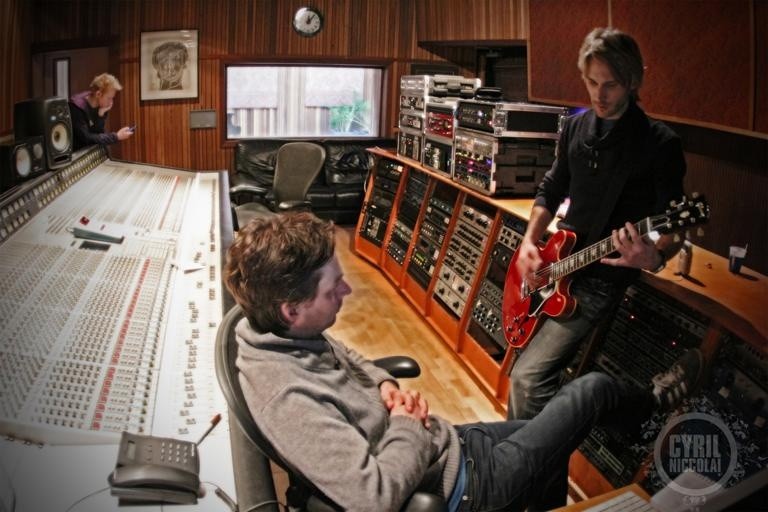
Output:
[228,136,396,226]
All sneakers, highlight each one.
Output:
[648,349,703,412]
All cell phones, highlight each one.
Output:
[129,128,134,131]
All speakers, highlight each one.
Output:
[0,135,48,193]
[14,96,75,171]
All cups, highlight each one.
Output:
[728,246,747,273]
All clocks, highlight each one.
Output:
[292,6,323,38]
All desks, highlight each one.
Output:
[350,145,767,512]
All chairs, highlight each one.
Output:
[228,142,326,233]
[215,304,449,512]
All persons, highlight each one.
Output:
[69,72,135,150]
[223,209,705,512]
[507,28,687,512]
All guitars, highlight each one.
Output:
[502,189,711,351]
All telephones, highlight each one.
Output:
[111,432,202,505]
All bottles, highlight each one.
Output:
[679,239,692,277]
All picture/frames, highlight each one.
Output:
[138,27,200,101]
